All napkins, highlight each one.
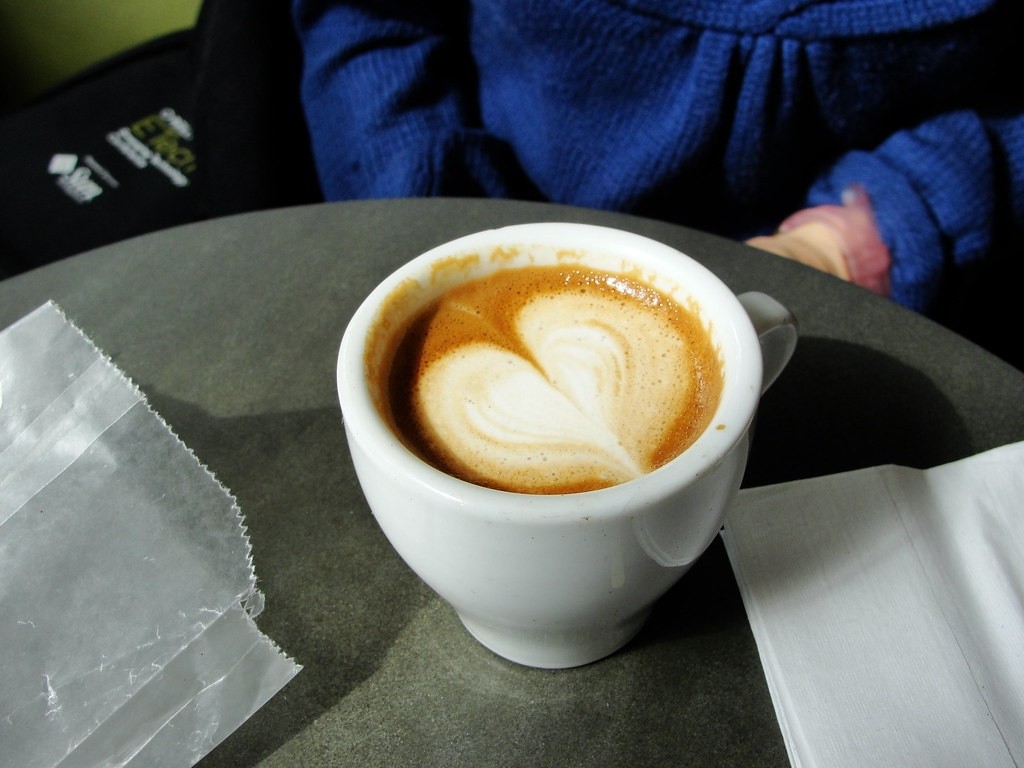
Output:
[716,438,1024,768]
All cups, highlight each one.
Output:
[335,222,800,669]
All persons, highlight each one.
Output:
[292,0,1024,323]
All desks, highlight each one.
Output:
[1,196,1024,766]
